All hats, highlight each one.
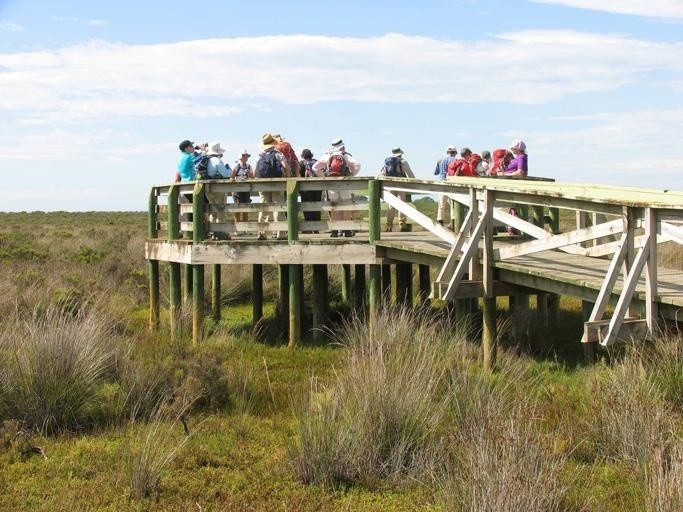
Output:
[257,132,279,151]
[508,139,526,152]
[178,140,194,151]
[205,143,226,156]
[389,147,404,157]
[239,149,251,157]
[330,138,344,152]
[300,149,313,159]
[446,145,457,154]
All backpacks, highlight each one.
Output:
[446,159,467,176]
[383,155,403,177]
[194,154,219,180]
[254,150,280,177]
[324,152,352,176]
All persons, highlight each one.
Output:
[432,139,528,236]
[312,139,361,239]
[297,149,323,233]
[252,133,300,238]
[231,150,254,237]
[377,146,415,232]
[174,141,233,240]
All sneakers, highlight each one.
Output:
[329,230,356,238]
